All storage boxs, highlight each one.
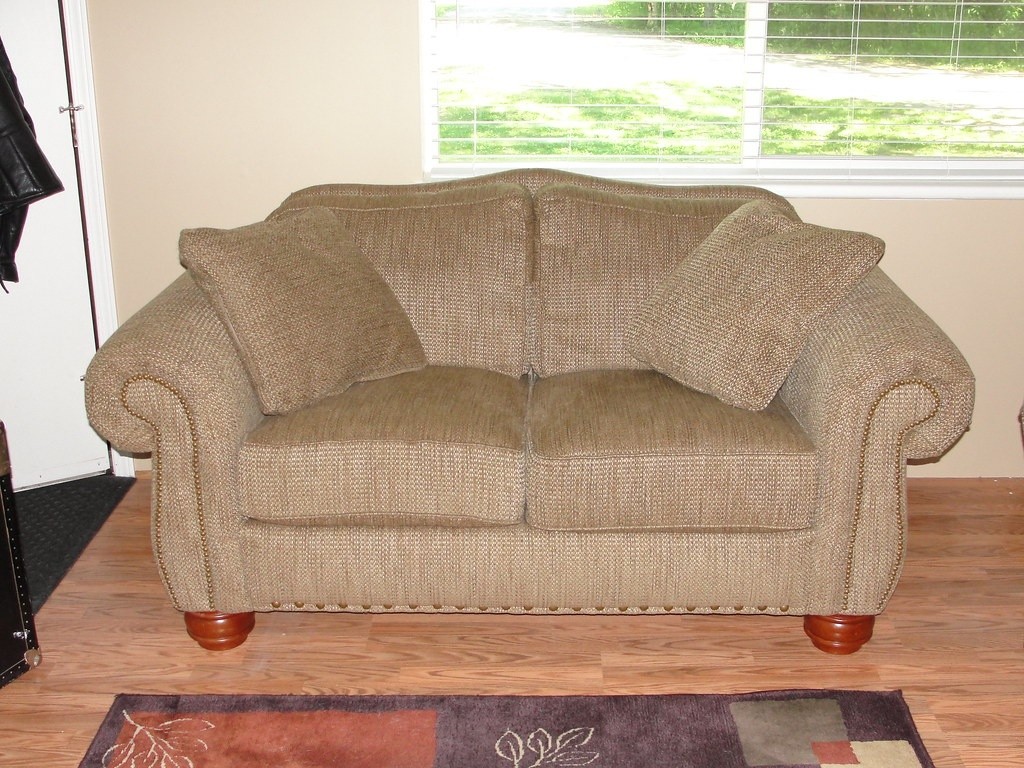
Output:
[0,420,42,690]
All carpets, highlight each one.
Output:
[78,687,935,768]
[13,475,136,618]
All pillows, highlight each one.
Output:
[179,206,428,417]
[624,200,886,412]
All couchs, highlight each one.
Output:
[84,168,977,655]
